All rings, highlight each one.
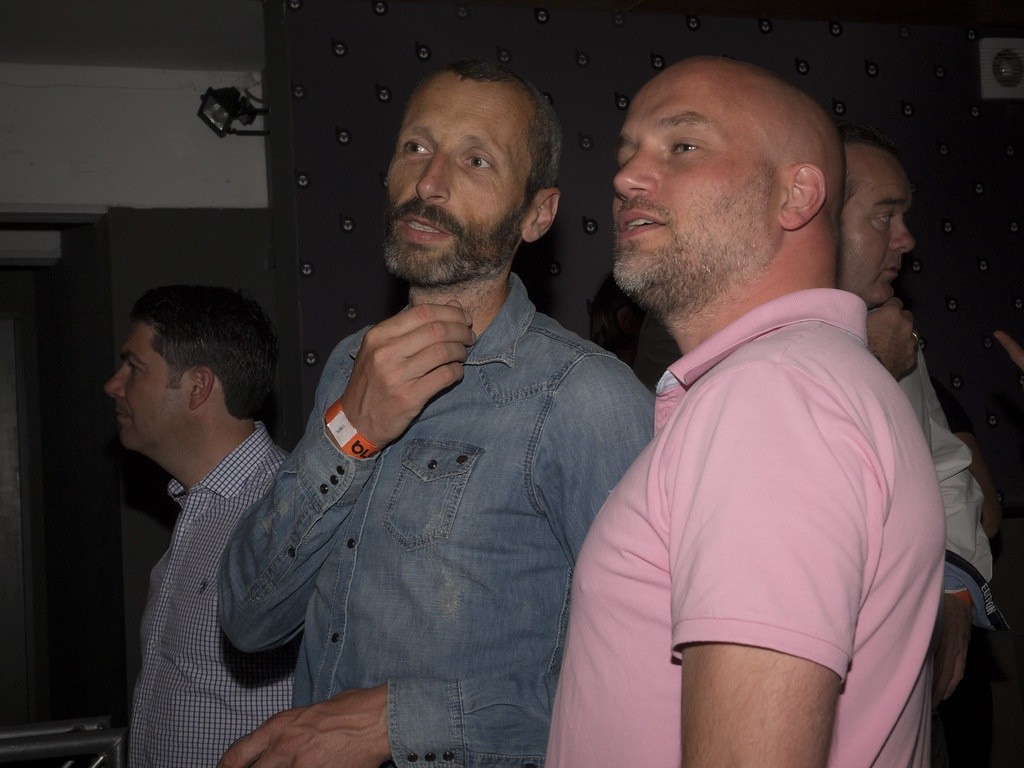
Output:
[912,332,920,343]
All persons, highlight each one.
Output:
[837,135,1024,767]
[543,53,941,768]
[102,283,297,768]
[217,58,656,768]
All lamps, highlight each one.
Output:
[979,38,1024,99]
[198,84,272,139]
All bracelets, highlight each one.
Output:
[326,399,380,459]
